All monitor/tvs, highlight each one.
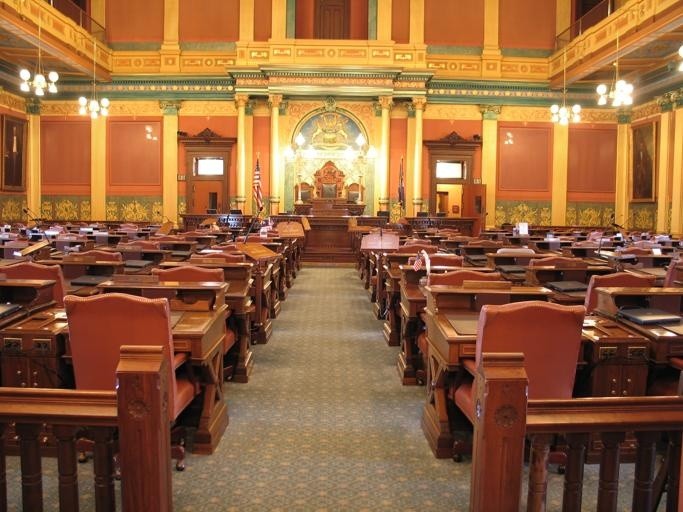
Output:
[206,209,216,214]
[437,212,446,217]
[417,211,427,217]
[230,209,242,214]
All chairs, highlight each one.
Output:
[62,292,188,475]
[295,160,365,205]
[454,305,587,477]
[370,220,668,386]
[0,210,285,379]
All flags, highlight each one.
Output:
[251,160,264,211]
[398,157,406,211]
[413,254,423,272]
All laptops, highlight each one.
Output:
[196,244,206,250]
[126,259,153,268]
[71,275,108,285]
[0,302,20,319]
[465,254,487,261]
[172,250,191,257]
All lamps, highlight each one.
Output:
[20,0,111,119]
[550,0,634,126]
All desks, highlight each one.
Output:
[0,280,53,456]
[32,281,230,455]
[595,288,683,461]
[422,283,642,459]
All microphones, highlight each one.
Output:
[22,208,51,246]
[27,207,39,224]
[243,207,263,243]
[158,211,169,221]
[620,213,635,225]
[597,213,615,256]
[379,229,383,255]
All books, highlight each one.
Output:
[125,259,152,268]
[466,252,489,261]
[0,302,24,320]
[170,248,191,257]
[70,274,108,286]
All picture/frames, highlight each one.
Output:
[629,120,657,204]
[0,112,28,193]
